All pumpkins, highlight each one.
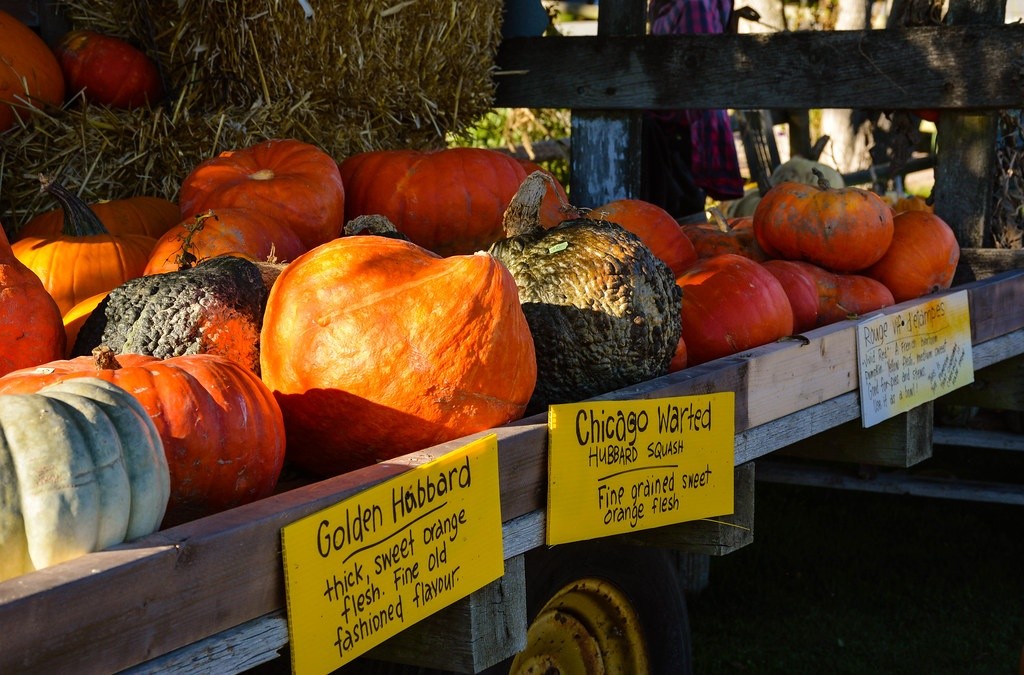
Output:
[0,11,961,581]
